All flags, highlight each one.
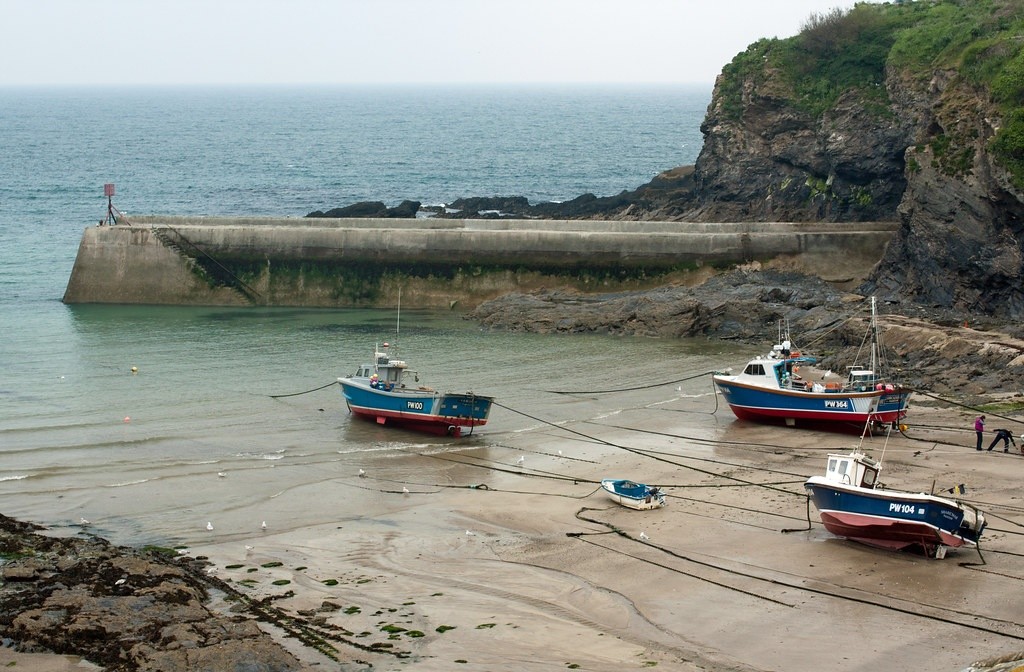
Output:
[821,369,831,380]
[948,485,965,494]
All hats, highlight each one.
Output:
[982,416,985,419]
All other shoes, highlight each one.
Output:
[977,448,982,450]
[1004,452,1008,453]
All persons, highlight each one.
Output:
[782,372,792,390]
[376,378,385,391]
[987,428,1018,453]
[975,415,986,451]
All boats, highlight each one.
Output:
[602,479,666,511]
[804,413,989,557]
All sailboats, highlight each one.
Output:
[712,296,915,428]
[337,287,495,438]
